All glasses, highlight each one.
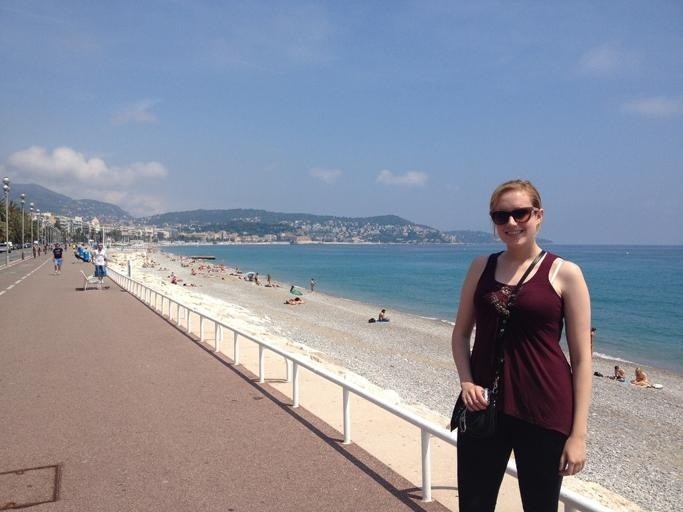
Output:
[491,207,539,226]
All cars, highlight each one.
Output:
[0,242,31,253]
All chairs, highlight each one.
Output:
[79,270,104,292]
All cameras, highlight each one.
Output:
[481,387,491,402]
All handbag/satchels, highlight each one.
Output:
[453,388,501,441]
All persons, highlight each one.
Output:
[615,366,625,380]
[591,327,596,335]
[379,309,389,320]
[52,242,317,304]
[452,179,592,512]
[632,368,648,386]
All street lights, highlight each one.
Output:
[2,176,11,267]
[19,192,26,260]
[36,208,61,252]
[29,201,34,254]
